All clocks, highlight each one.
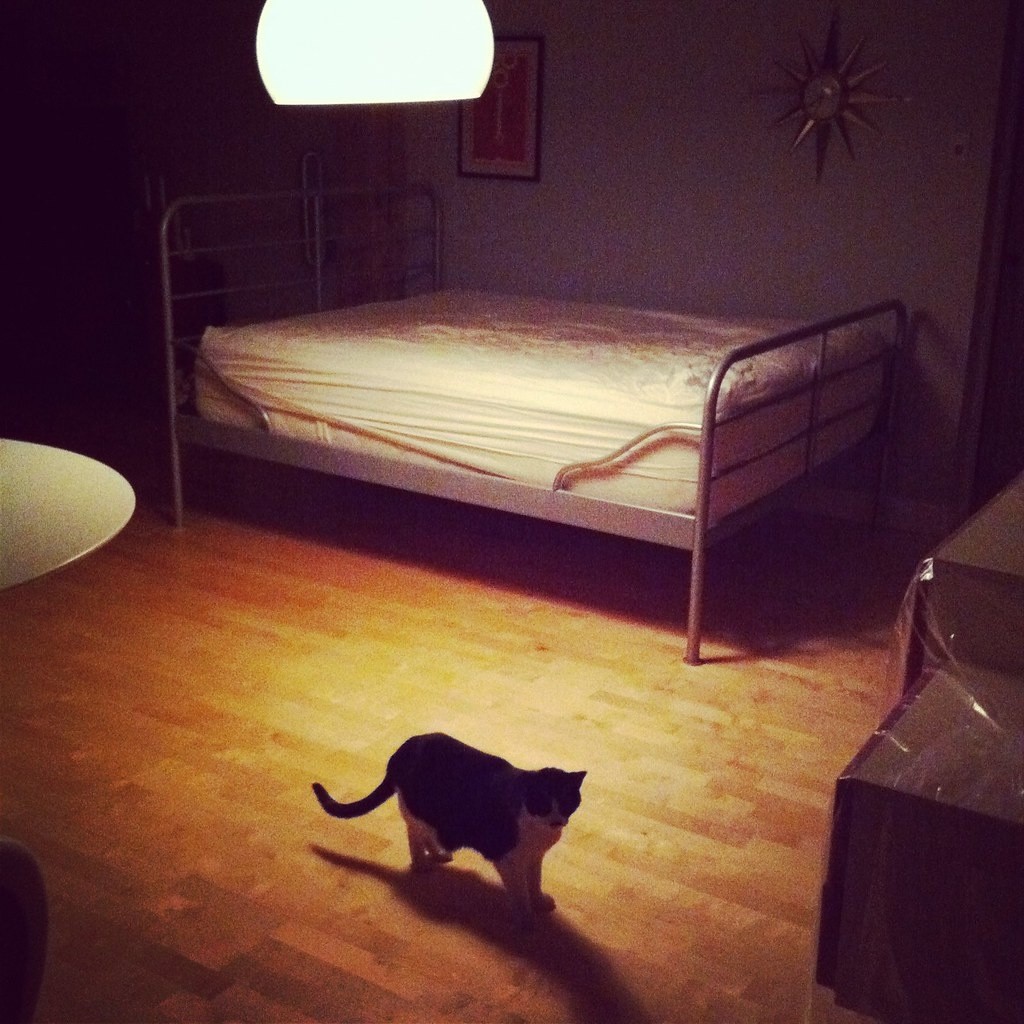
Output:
[754,0,915,187]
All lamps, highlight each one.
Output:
[255,0,495,106]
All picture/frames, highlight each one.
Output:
[456,34,544,183]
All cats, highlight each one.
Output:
[312,732,588,917]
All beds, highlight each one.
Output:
[159,178,907,665]
